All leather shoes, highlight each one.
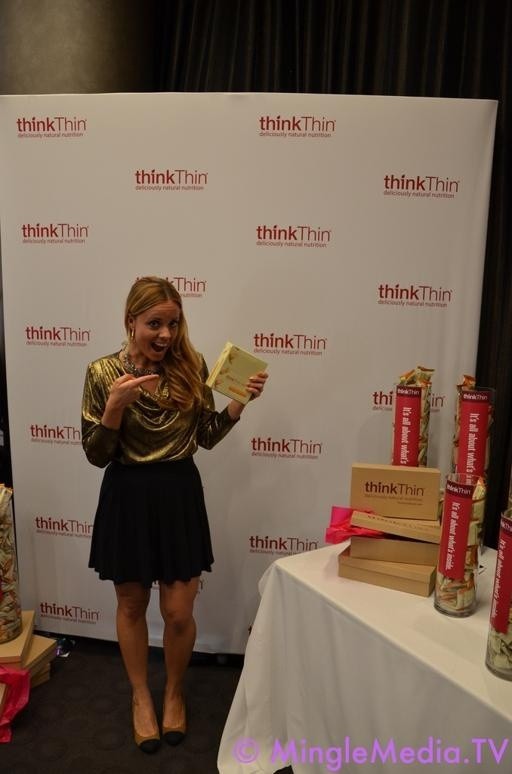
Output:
[130,701,162,755]
[162,701,186,747]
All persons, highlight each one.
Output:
[77,277,270,758]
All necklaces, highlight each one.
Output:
[119,339,167,377]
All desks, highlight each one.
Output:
[215,535,512,773]
